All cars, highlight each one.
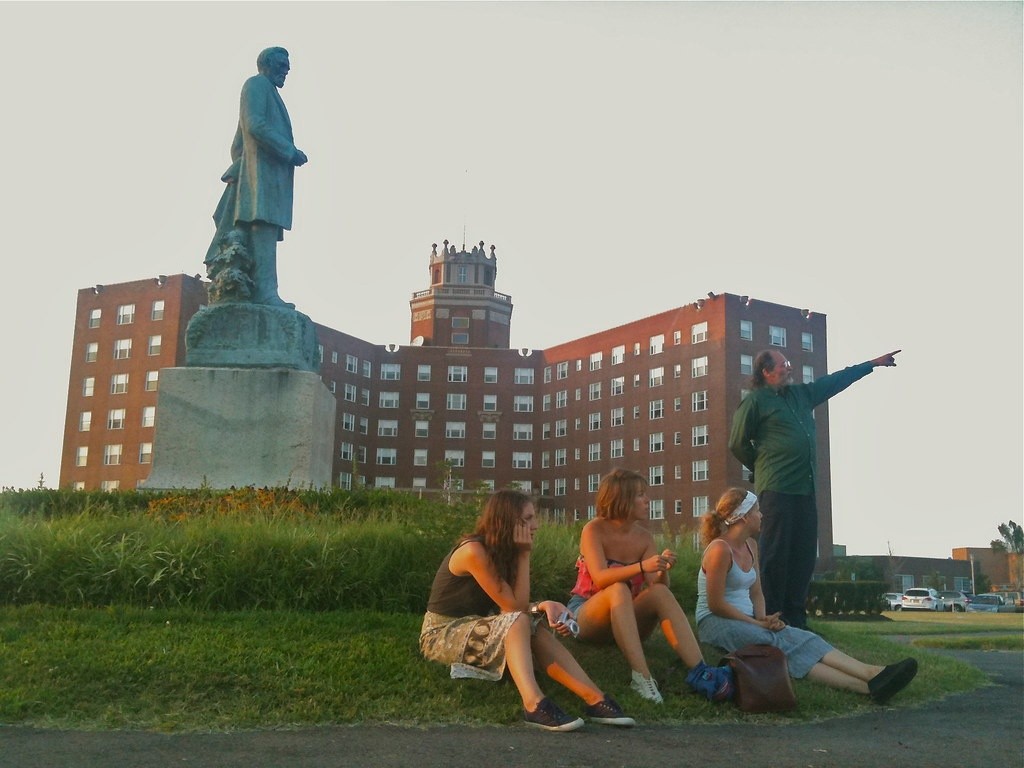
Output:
[939,591,1024,613]
[902,588,946,612]
[882,593,903,611]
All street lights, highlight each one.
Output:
[970,554,975,594]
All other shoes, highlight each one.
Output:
[802,627,824,639]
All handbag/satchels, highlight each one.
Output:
[717,643,797,713]
[682,658,733,705]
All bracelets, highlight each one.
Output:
[639,559,644,573]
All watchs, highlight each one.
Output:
[531,601,544,618]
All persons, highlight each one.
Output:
[729,349,901,636]
[419,490,636,730]
[696,487,918,704]
[567,468,706,705]
[203,47,308,310]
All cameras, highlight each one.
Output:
[556,611,579,638]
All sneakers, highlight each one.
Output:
[629,669,664,704]
[868,657,918,706]
[583,693,636,725]
[523,696,585,732]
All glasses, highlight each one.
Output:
[767,360,791,368]
